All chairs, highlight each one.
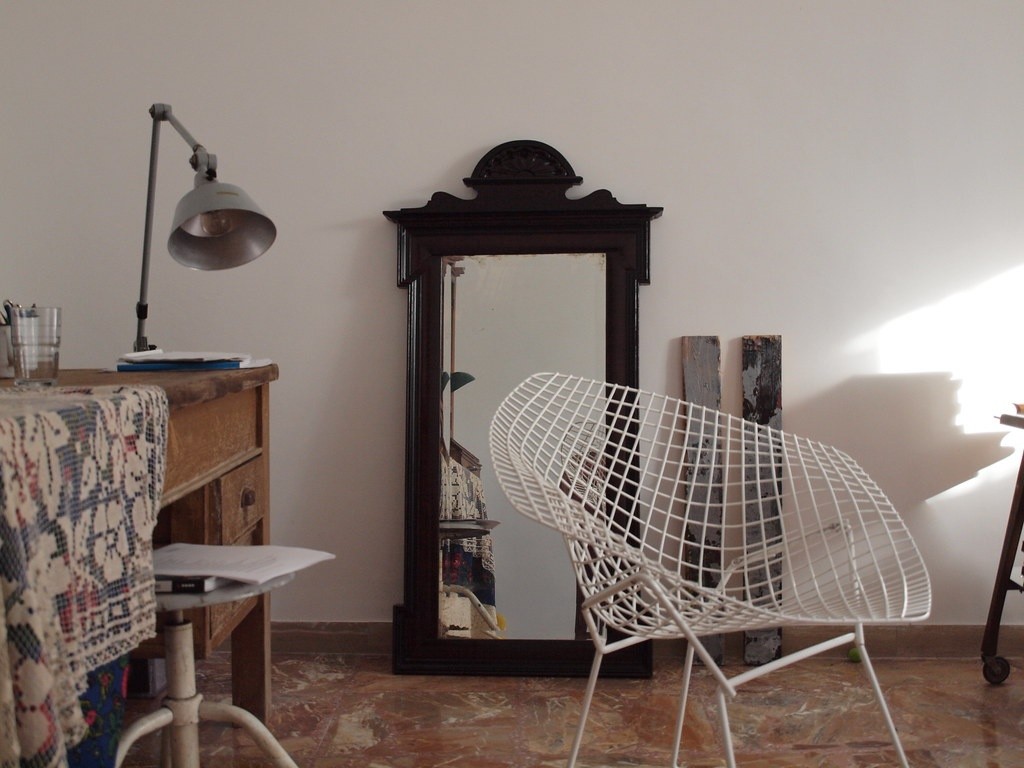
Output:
[490,372,932,768]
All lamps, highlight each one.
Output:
[134,105,278,353]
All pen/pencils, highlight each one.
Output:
[1,297,38,327]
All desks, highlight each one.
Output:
[0,362,279,768]
[439,437,502,637]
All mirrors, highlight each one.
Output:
[383,141,664,681]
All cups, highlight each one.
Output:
[10,307,62,386]
[0,324,16,378]
[17,315,38,371]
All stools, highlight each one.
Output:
[439,517,503,636]
[115,574,302,768]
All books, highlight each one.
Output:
[117,349,273,371]
[154,543,336,594]
[439,519,501,530]
